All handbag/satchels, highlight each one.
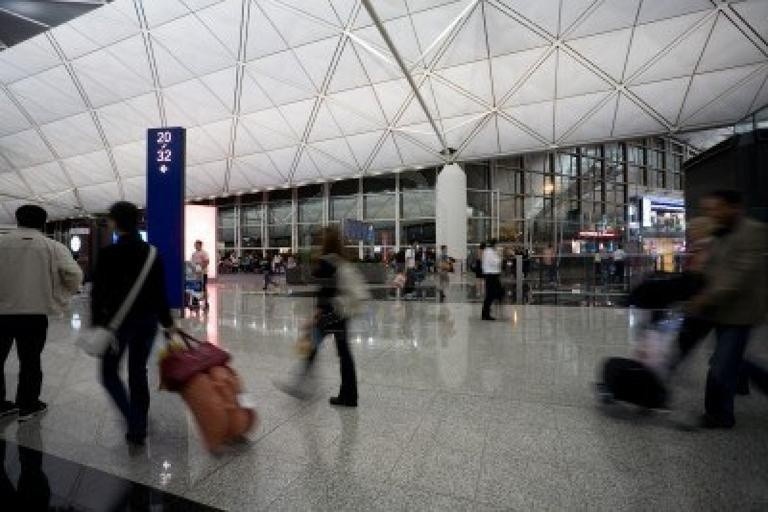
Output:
[158,327,230,392]
[74,327,114,356]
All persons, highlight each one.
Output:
[476,239,504,320]
[665,188,767,432]
[87,201,176,447]
[269,224,358,407]
[0,418,55,511]
[0,204,84,420]
[649,216,752,400]
[190,239,210,312]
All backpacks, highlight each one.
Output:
[322,253,371,320]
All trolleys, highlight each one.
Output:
[185,261,209,309]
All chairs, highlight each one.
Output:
[282,260,397,293]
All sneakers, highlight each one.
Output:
[128,437,146,458]
[18,399,49,422]
[0,401,19,416]
[697,412,736,430]
[482,316,496,320]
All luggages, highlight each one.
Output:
[179,361,255,457]
